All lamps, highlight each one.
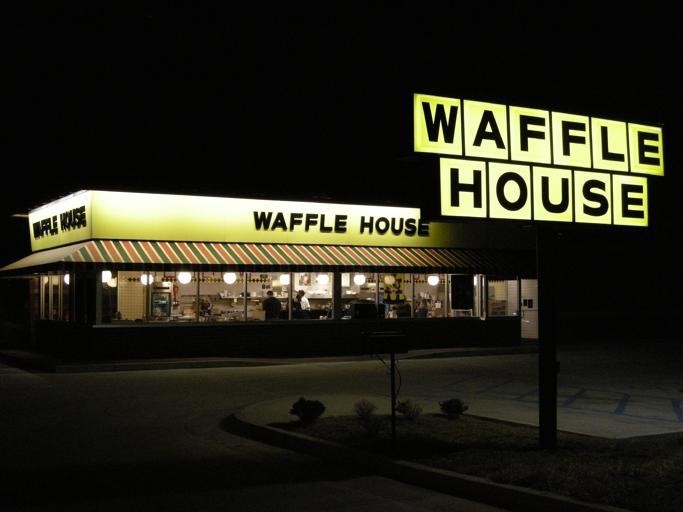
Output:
[176,271,192,285]
[101,270,118,288]
[426,273,440,288]
[140,270,153,286]
[384,275,396,286]
[222,271,237,285]
[278,272,366,288]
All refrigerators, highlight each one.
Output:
[145,281,174,324]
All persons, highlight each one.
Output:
[412,300,428,315]
[199,298,211,312]
[298,290,310,311]
[263,291,281,320]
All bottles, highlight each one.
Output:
[199,309,243,322]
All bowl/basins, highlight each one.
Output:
[250,290,288,298]
